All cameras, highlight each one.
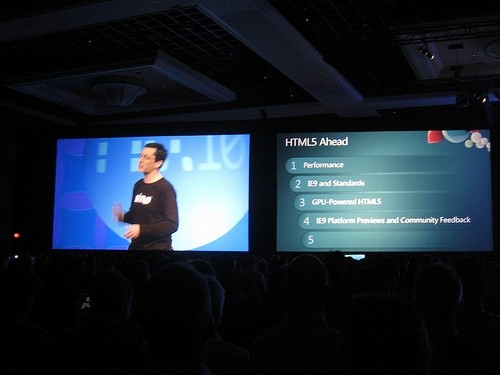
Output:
[342,253,368,264]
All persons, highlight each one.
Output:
[0,251,500,375]
[113,143,178,250]
